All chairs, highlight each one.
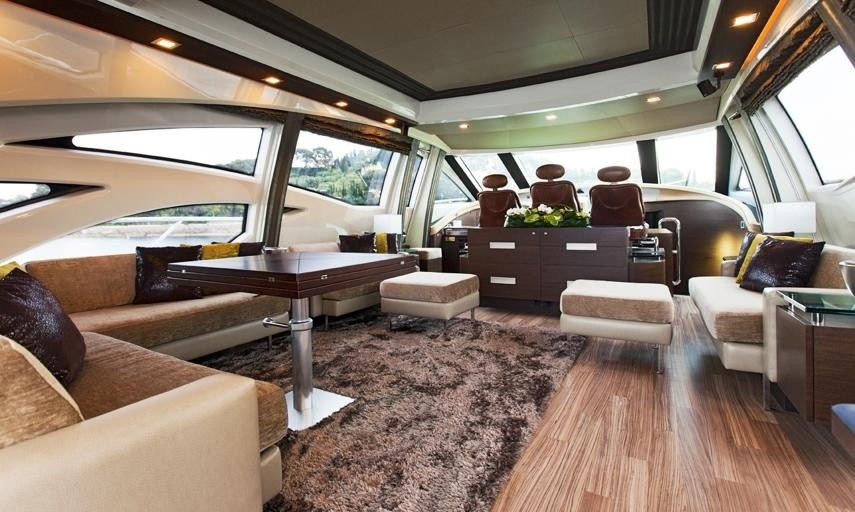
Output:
[588,164,647,225]
[529,164,581,211]
[477,174,521,228]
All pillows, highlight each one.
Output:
[337,233,377,253]
[375,232,387,253]
[387,233,399,254]
[0,260,27,277]
[211,241,266,256]
[178,241,242,298]
[1,267,87,389]
[133,243,203,302]
[733,230,828,293]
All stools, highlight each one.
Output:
[557,277,674,374]
[376,272,481,336]
[407,245,443,273]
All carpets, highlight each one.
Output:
[184,310,589,512]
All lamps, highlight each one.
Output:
[373,213,403,235]
[696,71,727,98]
[761,200,818,236]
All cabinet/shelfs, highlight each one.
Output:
[542,227,631,302]
[467,227,542,299]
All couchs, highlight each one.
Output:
[0,231,444,511]
[686,234,855,376]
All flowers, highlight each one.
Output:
[501,204,590,228]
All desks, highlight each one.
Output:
[165,250,422,429]
[629,250,666,285]
[776,289,855,426]
[647,227,674,296]
